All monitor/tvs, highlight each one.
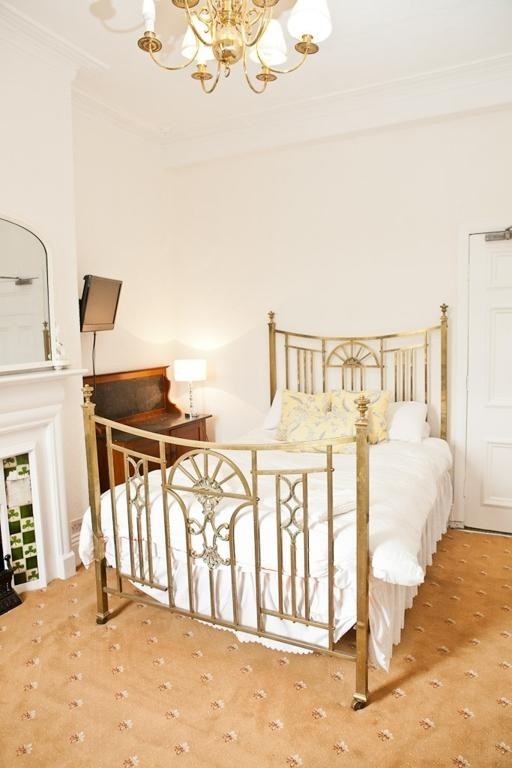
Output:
[82,274,123,335]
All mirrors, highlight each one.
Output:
[0,213,70,379]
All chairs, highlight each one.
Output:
[82,301,456,709]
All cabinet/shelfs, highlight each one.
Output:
[83,366,213,495]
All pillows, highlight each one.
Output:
[265,387,430,455]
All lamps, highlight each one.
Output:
[135,1,334,96]
[171,357,207,417]
